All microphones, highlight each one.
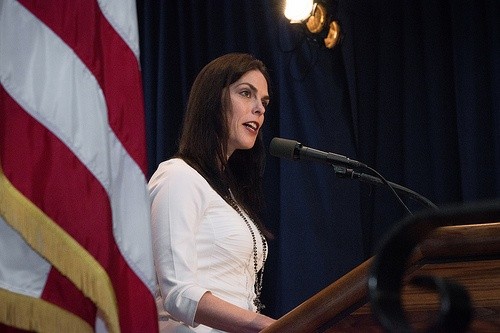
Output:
[269,139,366,171]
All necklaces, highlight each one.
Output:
[224,192,269,312]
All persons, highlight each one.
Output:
[147,53,278,333]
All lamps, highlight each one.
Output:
[276,0,342,50]
